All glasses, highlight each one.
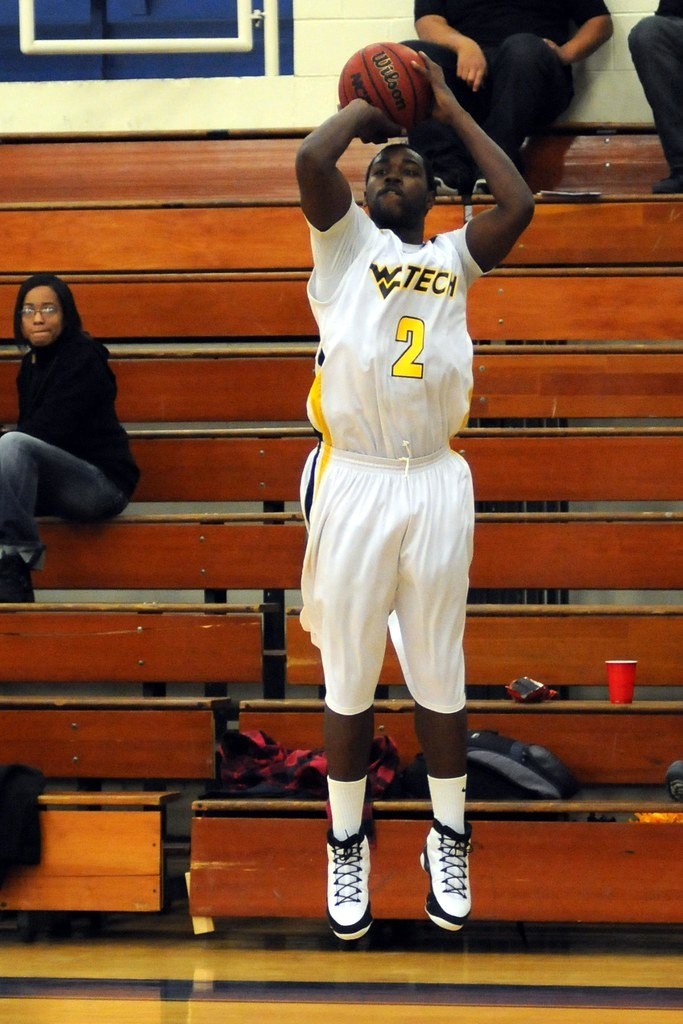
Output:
[22,304,61,316]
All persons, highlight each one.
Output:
[294,49,535,941]
[628,0,683,195]
[398,0,614,192]
[0,273,140,603]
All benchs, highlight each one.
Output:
[0,124,683,956]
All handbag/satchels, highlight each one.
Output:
[392,725,579,801]
[213,727,399,802]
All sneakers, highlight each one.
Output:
[0,553,35,604]
[420,818,473,931]
[326,824,374,941]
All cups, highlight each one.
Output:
[604,660,638,704]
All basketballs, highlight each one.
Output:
[337,40,431,132]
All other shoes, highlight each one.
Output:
[650,172,683,193]
[471,172,491,194]
[434,165,462,195]
